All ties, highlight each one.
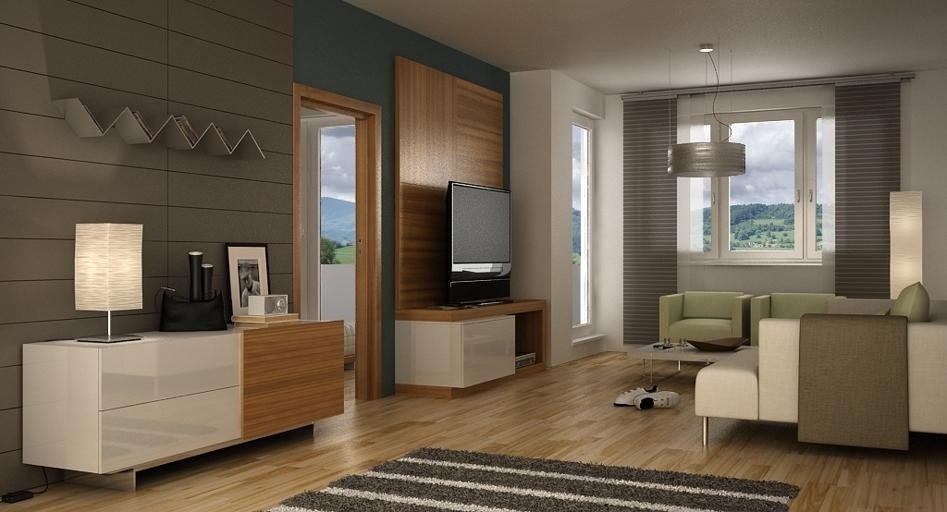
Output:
[445,180,513,305]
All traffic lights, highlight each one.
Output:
[874,281,929,322]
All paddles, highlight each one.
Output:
[251,446,802,512]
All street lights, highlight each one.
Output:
[154,286,228,330]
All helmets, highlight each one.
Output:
[686,337,750,352]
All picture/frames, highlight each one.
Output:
[626,341,758,386]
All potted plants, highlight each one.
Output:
[224,241,271,323]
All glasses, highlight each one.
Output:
[73,223,143,344]
[666,44,745,178]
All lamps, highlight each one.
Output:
[657,288,846,346]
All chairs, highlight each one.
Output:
[615,385,659,407]
[633,390,681,410]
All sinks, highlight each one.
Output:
[694,296,947,446]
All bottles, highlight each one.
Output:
[239,263,251,279]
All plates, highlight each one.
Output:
[393,297,549,400]
[18,317,344,491]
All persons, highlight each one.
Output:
[239,261,261,307]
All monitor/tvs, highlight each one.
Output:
[230,313,299,325]
[233,319,299,328]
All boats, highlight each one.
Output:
[248,293,290,317]
